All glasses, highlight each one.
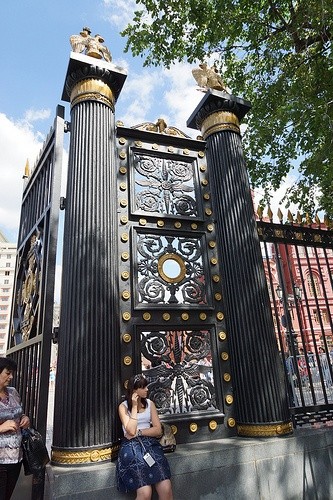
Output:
[134,374,143,384]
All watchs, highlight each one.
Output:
[138,429,142,437]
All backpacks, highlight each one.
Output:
[157,423,176,453]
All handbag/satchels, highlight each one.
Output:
[20,414,50,476]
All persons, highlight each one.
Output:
[0,357,30,500]
[116,374,173,500]
[199,63,223,90]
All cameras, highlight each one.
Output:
[143,452,155,467]
